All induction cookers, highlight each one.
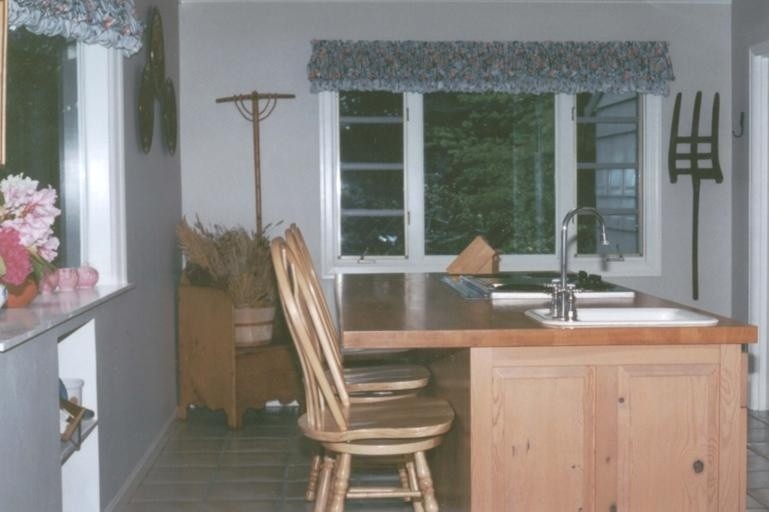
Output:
[461,270,637,301]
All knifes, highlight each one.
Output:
[480,220,511,250]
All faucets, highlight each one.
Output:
[558,204,609,318]
[524,304,720,326]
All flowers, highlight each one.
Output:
[1,173,64,286]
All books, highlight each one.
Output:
[441,271,491,305]
[458,267,569,293]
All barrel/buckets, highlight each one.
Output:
[233,307,276,347]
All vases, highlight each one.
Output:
[1,274,40,308]
[231,305,278,349]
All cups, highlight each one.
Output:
[36,262,102,297]
